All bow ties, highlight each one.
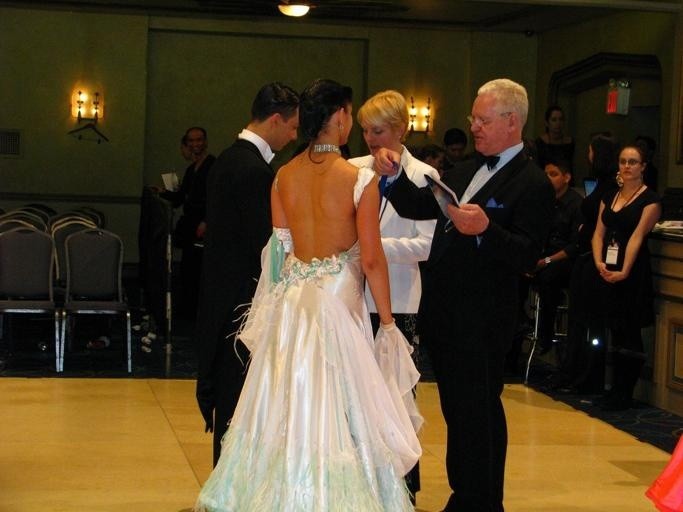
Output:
[474,152,500,170]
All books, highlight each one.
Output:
[424,173,460,218]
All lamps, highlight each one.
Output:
[66,86,109,144]
[278,5,310,17]
[405,95,437,138]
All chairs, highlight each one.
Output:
[523,289,570,384]
[0,202,132,374]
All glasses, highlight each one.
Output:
[467,112,512,128]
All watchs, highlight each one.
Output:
[544,256,551,264]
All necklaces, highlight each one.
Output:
[312,145,342,154]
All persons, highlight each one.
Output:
[346,89,440,505]
[197,83,299,468]
[192,77,424,512]
[634,135,659,191]
[579,144,663,411]
[529,105,567,170]
[418,144,445,176]
[150,127,218,325]
[538,159,594,395]
[372,79,555,512]
[576,138,620,213]
[441,128,468,170]
